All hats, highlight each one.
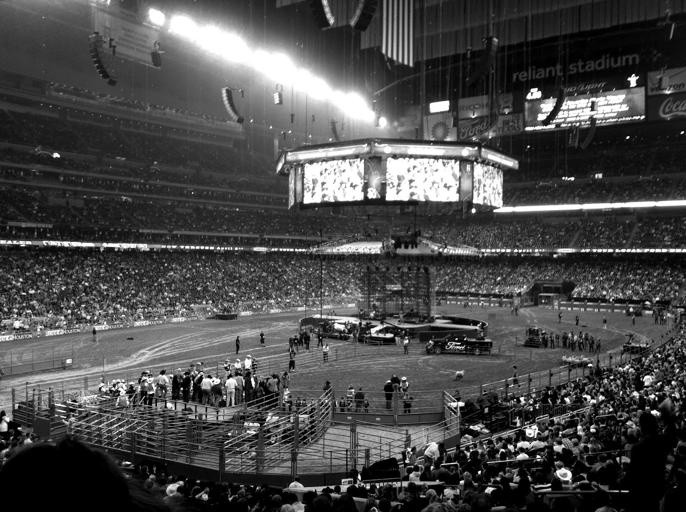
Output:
[166,486,177,496]
[247,355,251,357]
[526,429,534,437]
[556,468,572,481]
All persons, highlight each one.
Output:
[1,109,686,511]
